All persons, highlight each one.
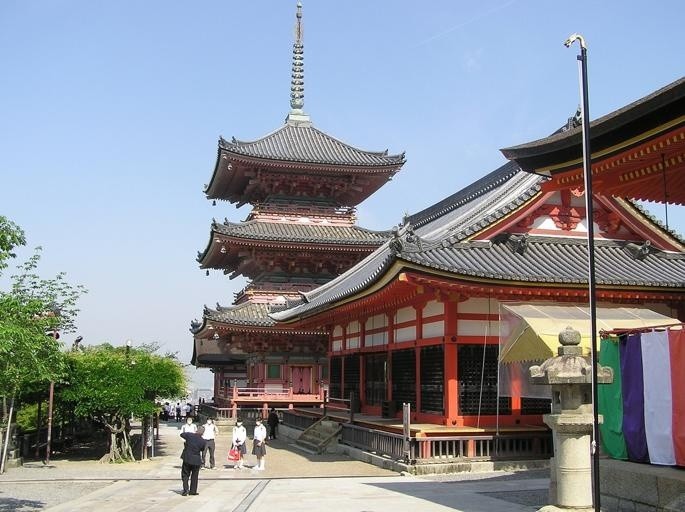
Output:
[180,417,197,448]
[267,407,279,439]
[179,424,207,495]
[201,416,219,470]
[160,396,202,421]
[230,416,247,468]
[251,417,267,470]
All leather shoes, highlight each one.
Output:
[182,491,199,496]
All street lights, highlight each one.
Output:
[45,330,60,466]
[73,334,85,349]
[124,339,132,359]
[561,29,603,512]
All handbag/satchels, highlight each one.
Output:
[228,450,240,461]
